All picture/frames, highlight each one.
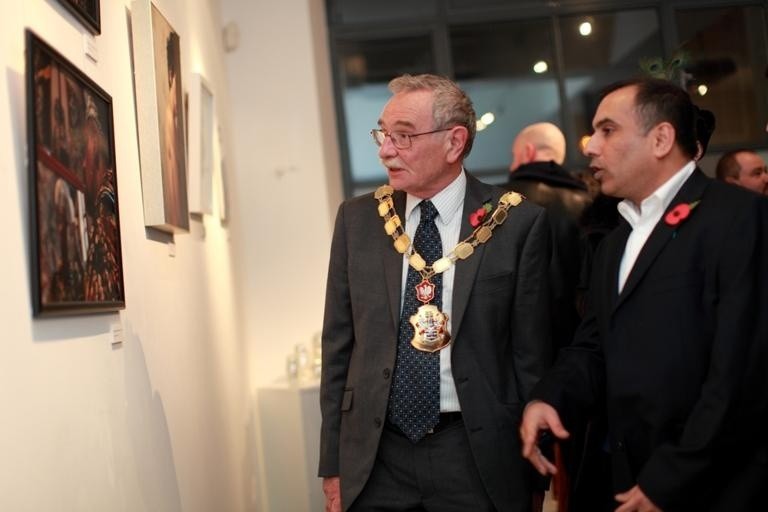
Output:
[50,0,105,36]
[184,71,214,218]
[21,25,126,321]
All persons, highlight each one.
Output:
[495,122,593,359]
[518,77,768,512]
[716,149,768,195]
[163,30,188,229]
[317,72,551,512]
[692,105,716,162]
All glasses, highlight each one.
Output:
[368,128,451,149]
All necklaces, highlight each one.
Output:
[372,181,528,355]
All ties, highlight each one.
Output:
[389,200,444,443]
